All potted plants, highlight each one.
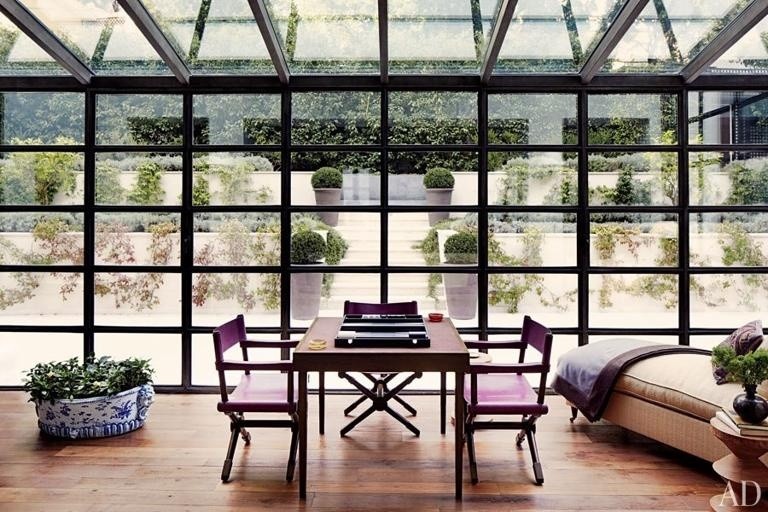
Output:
[443,234,478,320]
[291,231,327,319]
[24,355,156,438]
[311,167,344,226]
[712,344,768,424]
[321,229,350,297]
[423,168,454,226]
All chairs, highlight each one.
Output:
[338,301,420,436]
[462,315,553,483]
[213,314,300,482]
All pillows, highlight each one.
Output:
[713,320,764,384]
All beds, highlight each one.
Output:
[552,337,768,464]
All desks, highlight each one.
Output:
[710,417,768,512]
[293,316,470,501]
